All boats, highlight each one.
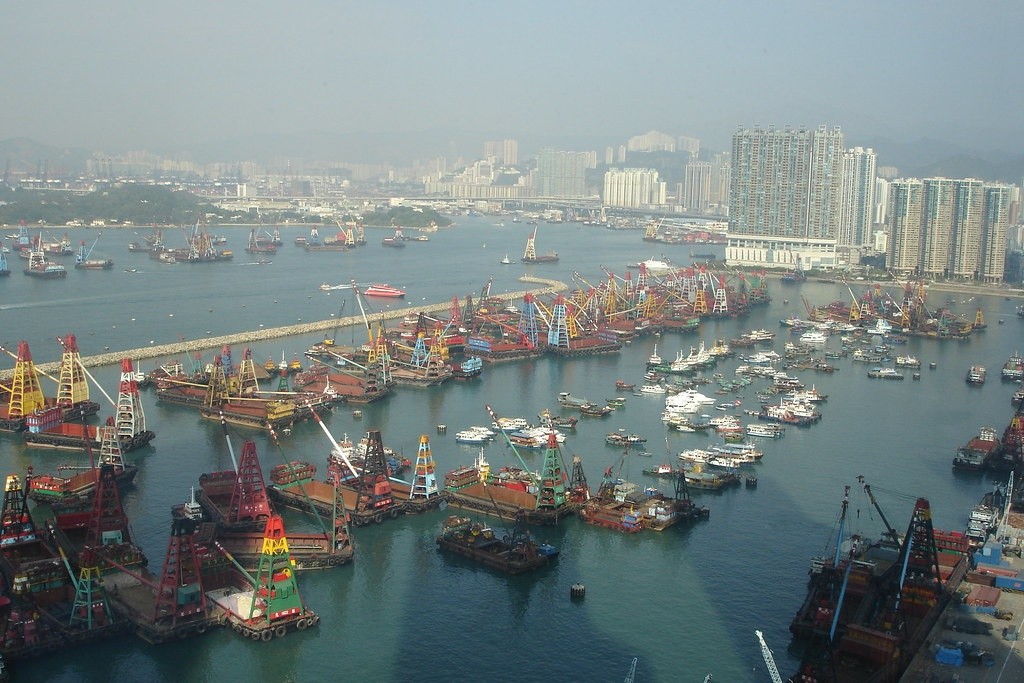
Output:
[1,265,1024,683]
[0,220,561,280]
